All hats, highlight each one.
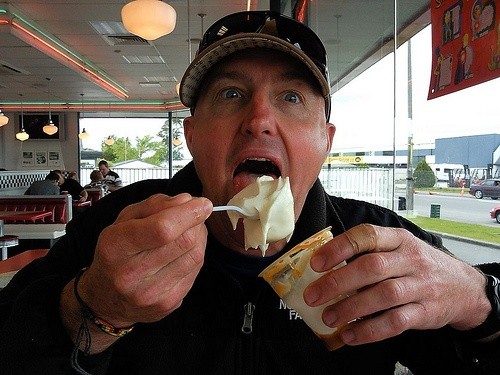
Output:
[178,10,332,125]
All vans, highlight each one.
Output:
[428,163,465,182]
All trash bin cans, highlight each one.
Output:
[398,196,406,210]
[431,204,441,218]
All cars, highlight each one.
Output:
[81,146,103,160]
[490,206,500,224]
[468,179,500,200]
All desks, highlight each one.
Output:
[0,211,52,224]
[73,201,91,208]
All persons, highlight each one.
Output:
[24,173,60,194]
[0,10,500,375]
[84,170,110,197]
[50,170,88,203]
[98,160,123,192]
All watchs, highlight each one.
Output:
[460,269,500,338]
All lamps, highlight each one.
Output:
[42,77,58,135]
[121,0,177,41]
[105,102,114,145]
[78,94,88,138]
[0,109,9,128]
[173,97,181,146]
[15,94,29,142]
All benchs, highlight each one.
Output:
[86,189,104,202]
[0,195,73,249]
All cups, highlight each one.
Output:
[256,225,370,353]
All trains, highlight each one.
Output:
[322,142,435,168]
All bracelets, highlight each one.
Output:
[70,266,136,375]
